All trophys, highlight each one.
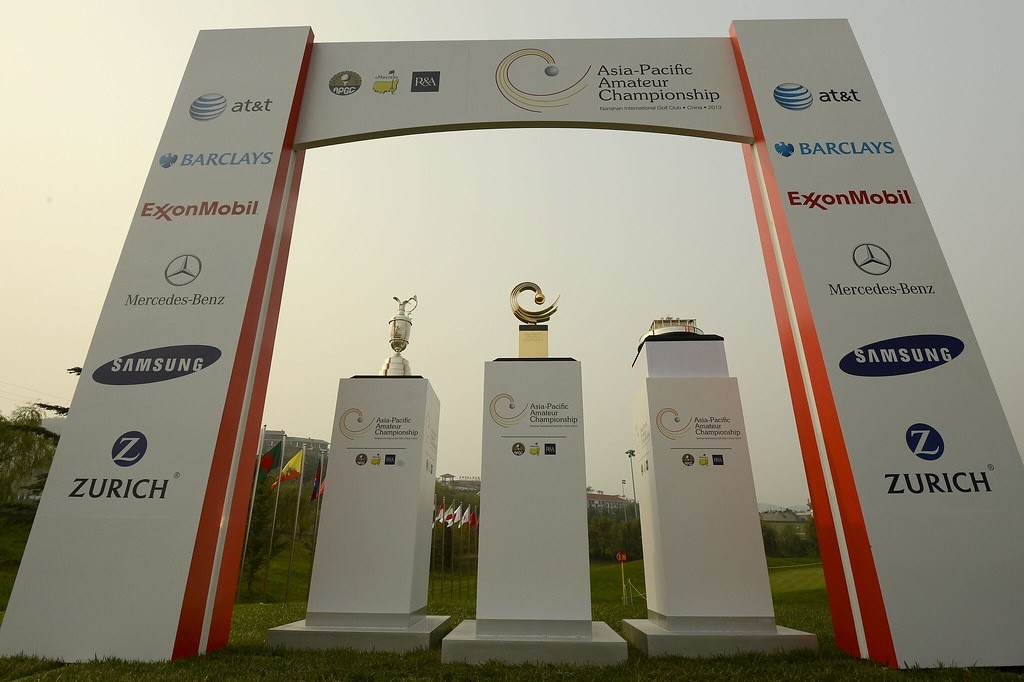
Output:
[510,282,560,359]
[383,295,418,376]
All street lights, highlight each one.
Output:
[626,449,638,518]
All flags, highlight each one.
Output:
[271,449,302,488]
[432,502,435,529]
[320,476,326,495]
[444,502,455,528]
[258,440,281,482]
[435,500,443,523]
[466,511,475,531]
[309,458,321,503]
[458,507,469,528]
[453,507,461,523]
